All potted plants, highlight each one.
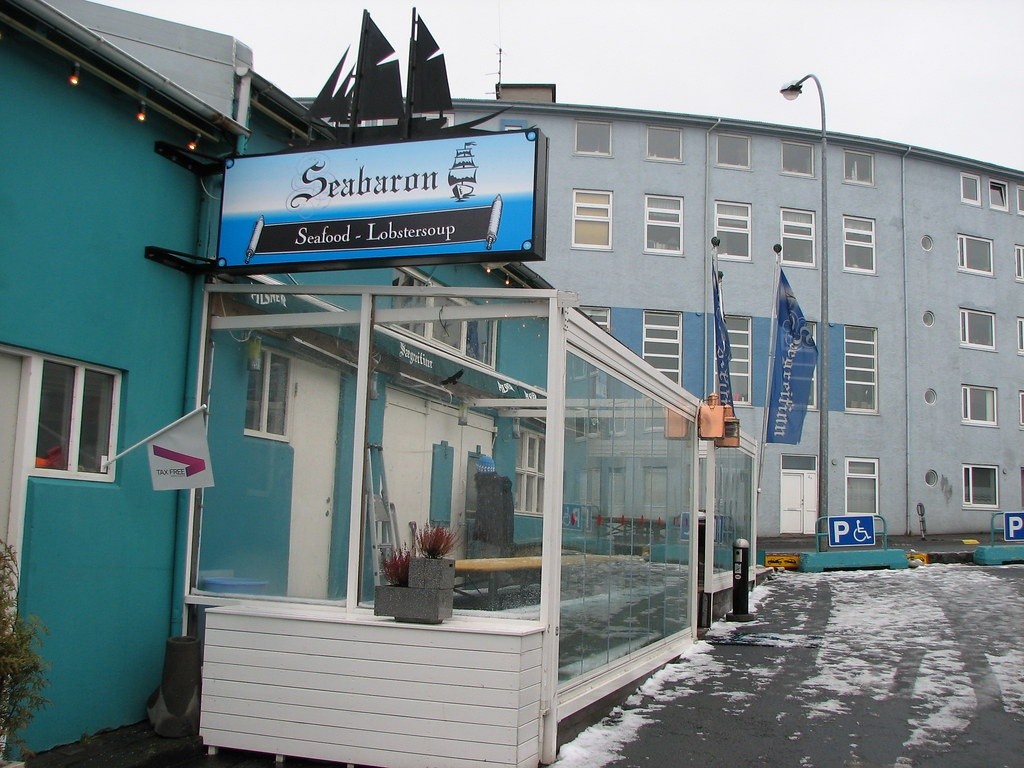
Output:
[373,519,466,625]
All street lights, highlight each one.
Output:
[778,74,833,549]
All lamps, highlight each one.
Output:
[715,404,740,448]
[698,393,725,440]
